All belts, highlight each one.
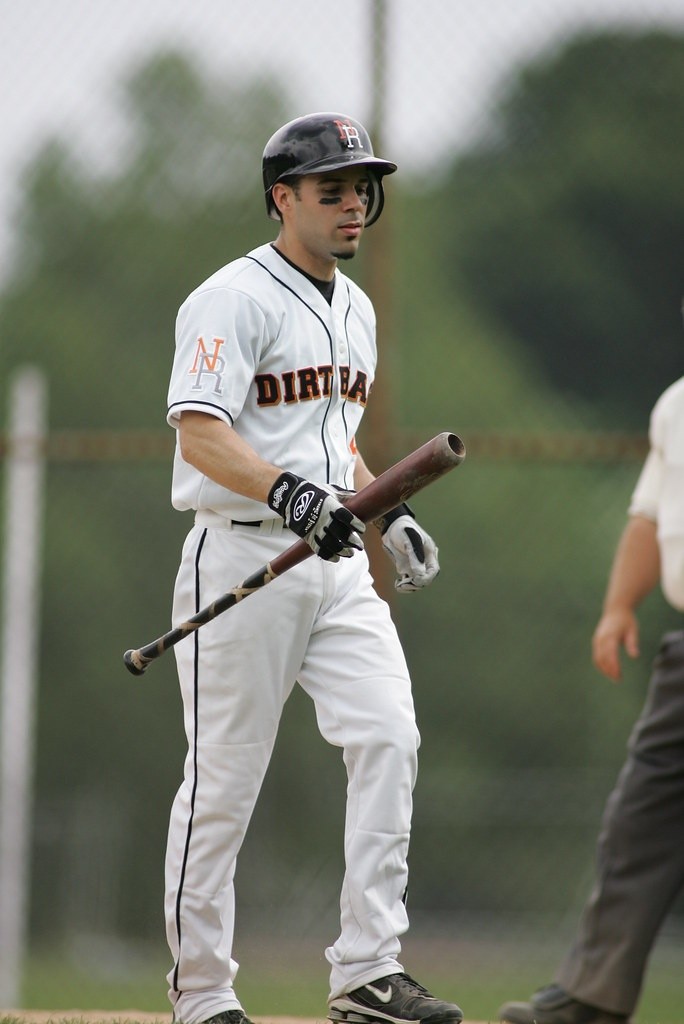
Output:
[232,520,263,528]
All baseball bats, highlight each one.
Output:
[122,429,468,679]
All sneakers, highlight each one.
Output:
[200,1009,255,1024]
[328,971,463,1024]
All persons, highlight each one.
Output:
[161,110,464,1022]
[495,372,684,1021]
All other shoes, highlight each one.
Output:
[498,982,628,1024]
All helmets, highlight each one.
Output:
[263,112,397,229]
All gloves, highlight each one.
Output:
[374,506,440,595]
[268,471,367,563]
